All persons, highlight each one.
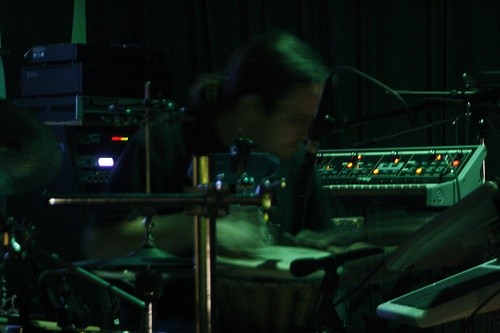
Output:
[84,32,331,266]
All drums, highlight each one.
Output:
[212,245,332,333]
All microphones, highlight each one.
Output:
[304,77,332,160]
[291,246,385,277]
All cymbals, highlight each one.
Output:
[72,245,195,269]
